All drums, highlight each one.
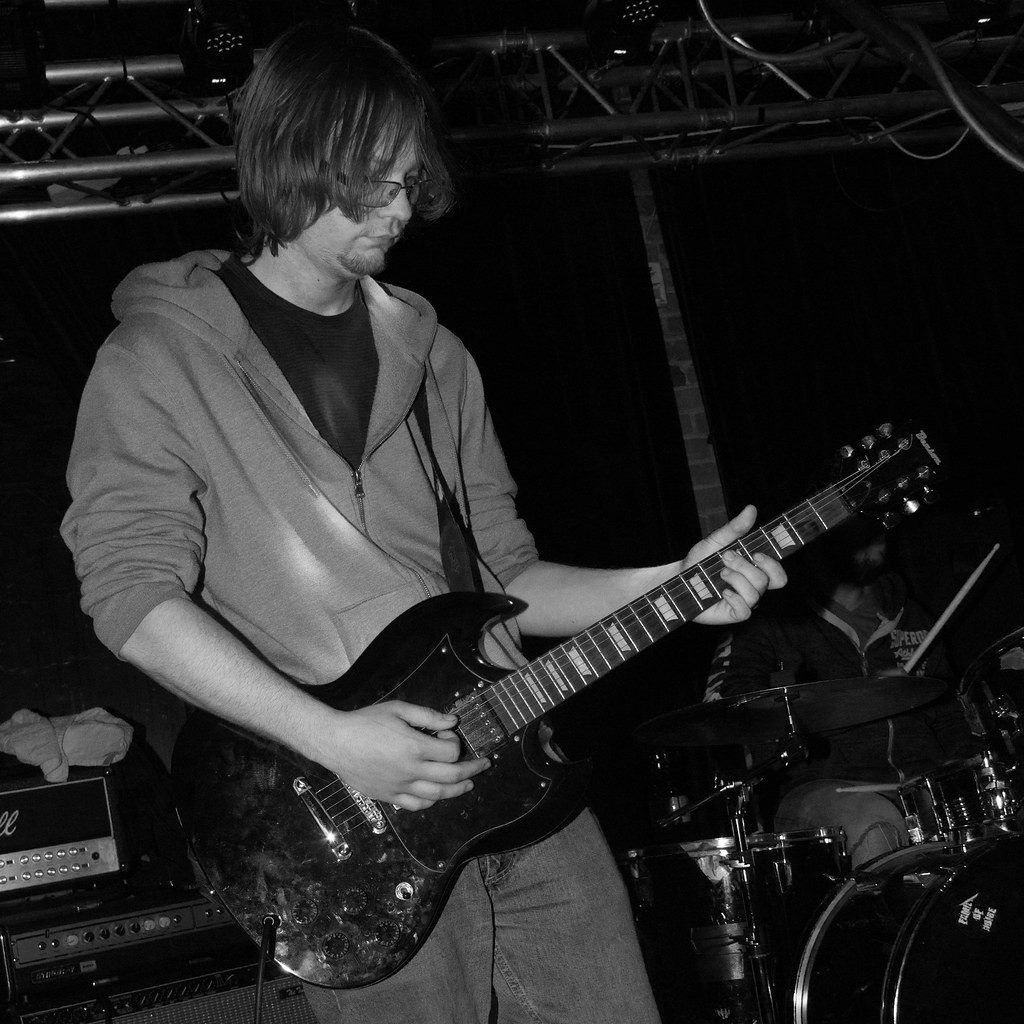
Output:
[958,622,1024,789]
[899,753,1015,841]
[785,837,1023,1023]
[615,823,849,1024]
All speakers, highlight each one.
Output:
[0,957,320,1024]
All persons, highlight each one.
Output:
[58,23,790,1024]
[701,468,1024,872]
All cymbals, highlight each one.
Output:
[636,672,945,748]
[663,776,755,831]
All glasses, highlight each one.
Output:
[330,167,437,208]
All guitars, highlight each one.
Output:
[151,420,955,991]
